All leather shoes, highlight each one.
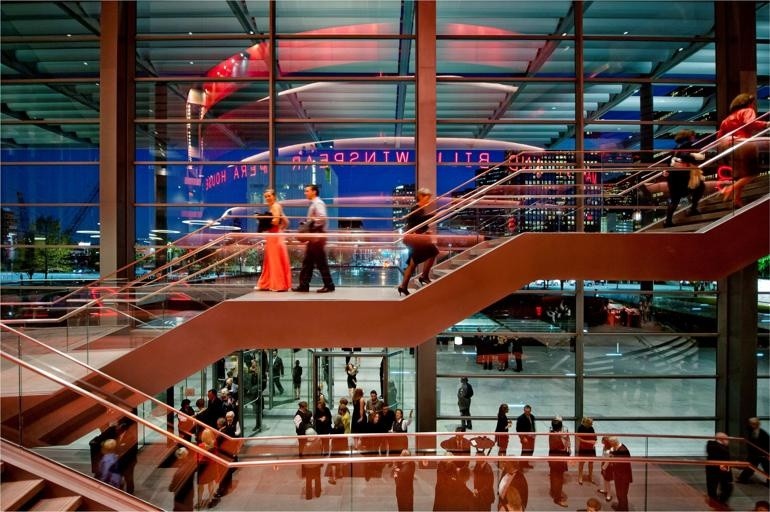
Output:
[662,220,677,228]
[292,286,309,292]
[687,208,701,216]
[316,284,334,292]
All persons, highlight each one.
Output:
[290,183,335,292]
[253,188,293,292]
[663,130,706,227]
[706,432,733,502]
[717,93,770,208]
[755,501,770,512]
[734,418,770,489]
[398,188,440,296]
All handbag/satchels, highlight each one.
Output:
[671,156,689,168]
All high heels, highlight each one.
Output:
[723,186,743,207]
[398,287,410,296]
[419,277,432,286]
